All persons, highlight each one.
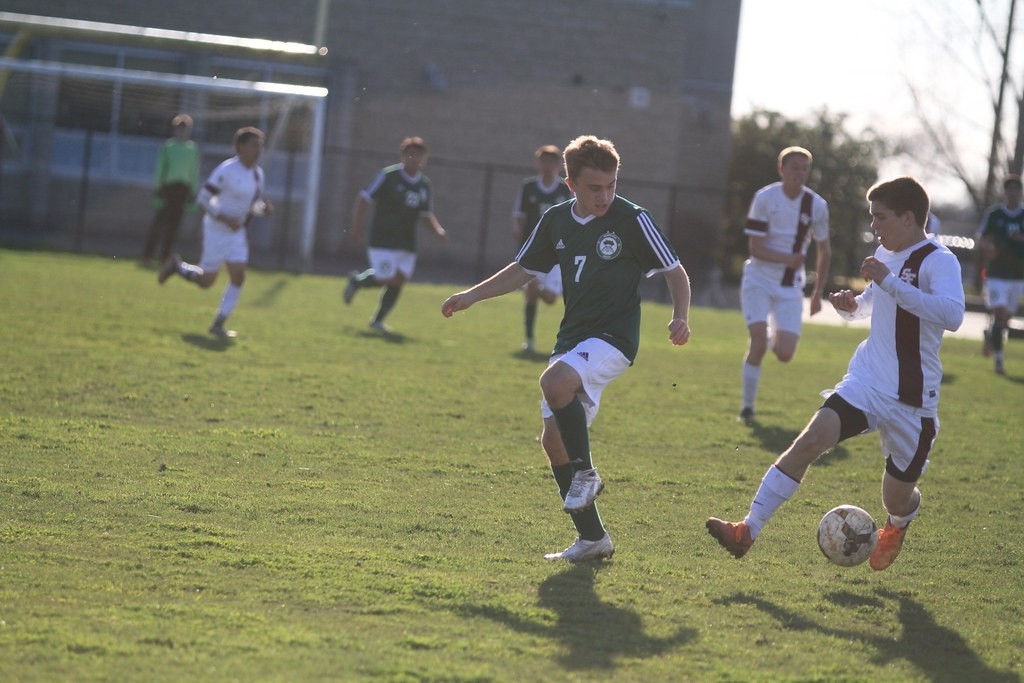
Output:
[973,175,1024,374]
[511,145,574,355]
[137,114,200,270]
[159,127,271,340]
[441,134,691,562]
[342,137,447,335]
[706,177,966,570]
[740,146,831,424]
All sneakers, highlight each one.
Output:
[563,467,603,513]
[544,532,615,563]
[705,517,753,558]
[870,515,911,571]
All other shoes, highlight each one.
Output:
[370,321,390,332]
[740,407,753,427]
[158,254,179,282]
[345,271,359,302]
[984,331,992,356]
[210,326,235,340]
[996,363,1003,374]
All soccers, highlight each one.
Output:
[817,504,880,568]
